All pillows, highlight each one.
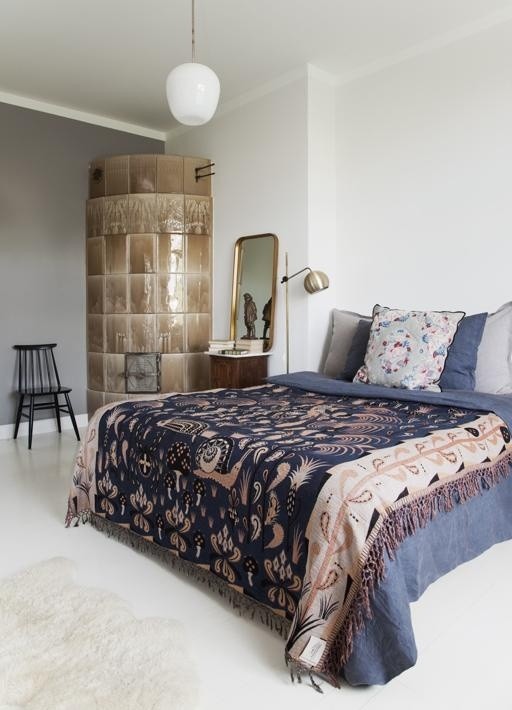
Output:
[358,301,464,394]
[340,313,486,389]
[321,303,511,395]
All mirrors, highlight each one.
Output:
[229,232,278,351]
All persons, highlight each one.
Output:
[243,293,257,339]
[262,297,272,338]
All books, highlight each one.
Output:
[208,340,264,357]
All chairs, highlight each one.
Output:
[12,342,81,450]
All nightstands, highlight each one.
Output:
[205,351,271,390]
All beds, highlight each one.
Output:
[70,301,511,692]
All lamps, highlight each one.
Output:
[281,252,330,375]
[163,0,222,129]
